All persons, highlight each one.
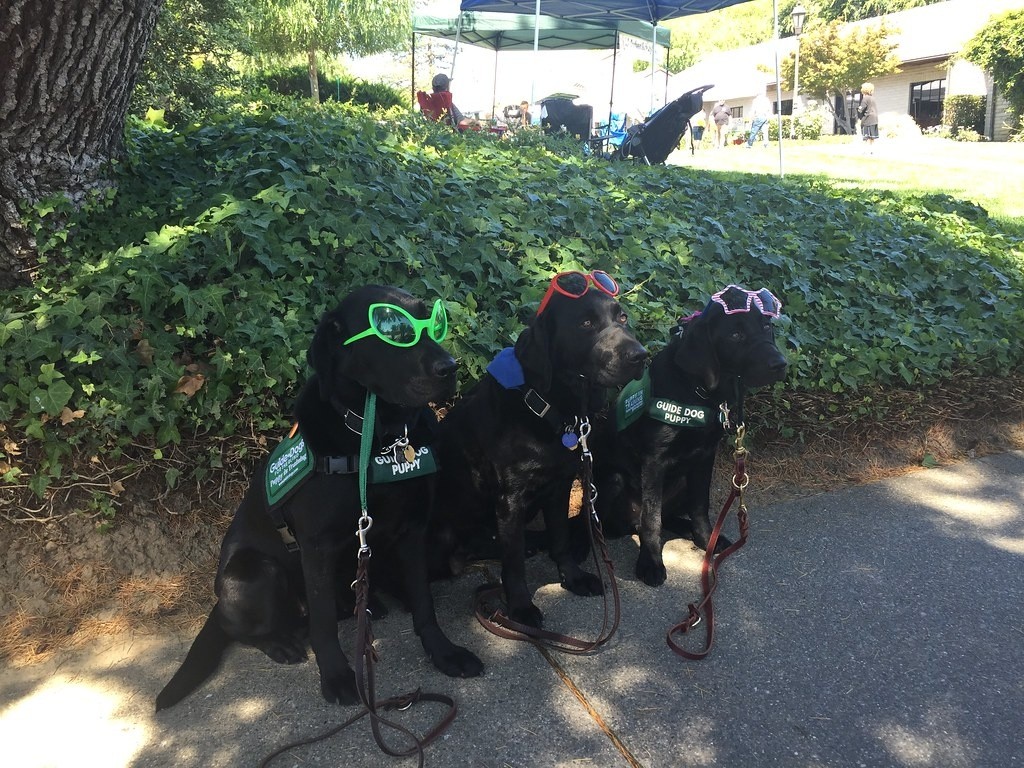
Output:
[712,97,732,149]
[744,92,771,148]
[517,101,532,125]
[419,73,482,133]
[857,83,880,157]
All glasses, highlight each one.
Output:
[537,271,620,318]
[342,298,451,348]
[712,285,782,318]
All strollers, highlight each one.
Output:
[610,84,714,168]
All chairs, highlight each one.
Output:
[416,89,506,139]
[538,97,617,157]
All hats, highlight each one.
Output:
[432,74,454,87]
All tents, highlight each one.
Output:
[411,5,671,154]
[449,0,782,174]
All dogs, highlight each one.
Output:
[434,289,650,630]
[593,291,786,586]
[155,285,487,721]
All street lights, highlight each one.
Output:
[789,4,807,140]
